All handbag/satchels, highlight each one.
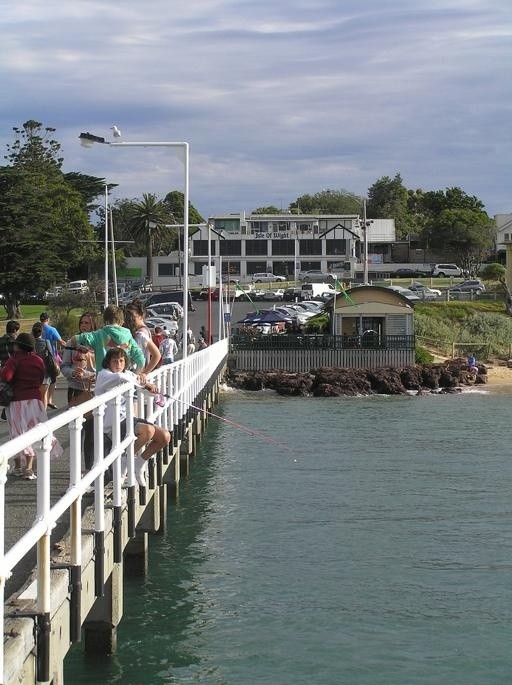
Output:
[44,338,54,377]
[0,358,19,406]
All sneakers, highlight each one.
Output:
[24,468,37,480]
[6,466,22,477]
[135,454,148,487]
[121,452,127,486]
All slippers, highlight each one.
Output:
[47,403,58,409]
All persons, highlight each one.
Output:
[468,354,478,377]
[1,300,209,489]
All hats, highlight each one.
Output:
[160,330,170,336]
[9,333,35,348]
[40,313,48,320]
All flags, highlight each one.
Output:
[336,279,354,305]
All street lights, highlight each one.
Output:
[78,132,190,359]
[326,189,369,286]
[180,248,222,341]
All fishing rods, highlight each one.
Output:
[142,384,295,454]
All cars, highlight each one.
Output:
[389,268,426,279]
[222,270,337,284]
[238,300,325,336]
[385,280,486,300]
[132,290,196,333]
[188,282,341,302]
[0,279,153,307]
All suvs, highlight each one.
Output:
[431,263,470,278]
